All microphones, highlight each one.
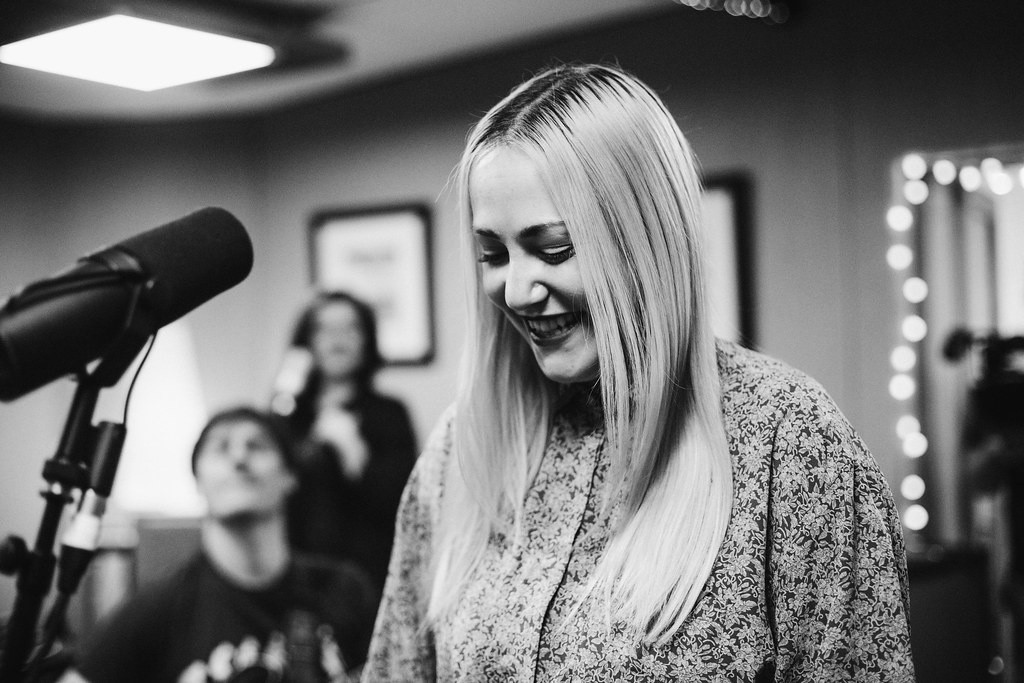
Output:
[0,208,255,404]
[273,348,312,416]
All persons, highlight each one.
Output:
[265,291,425,605]
[360,62,917,683]
[52,404,373,681]
